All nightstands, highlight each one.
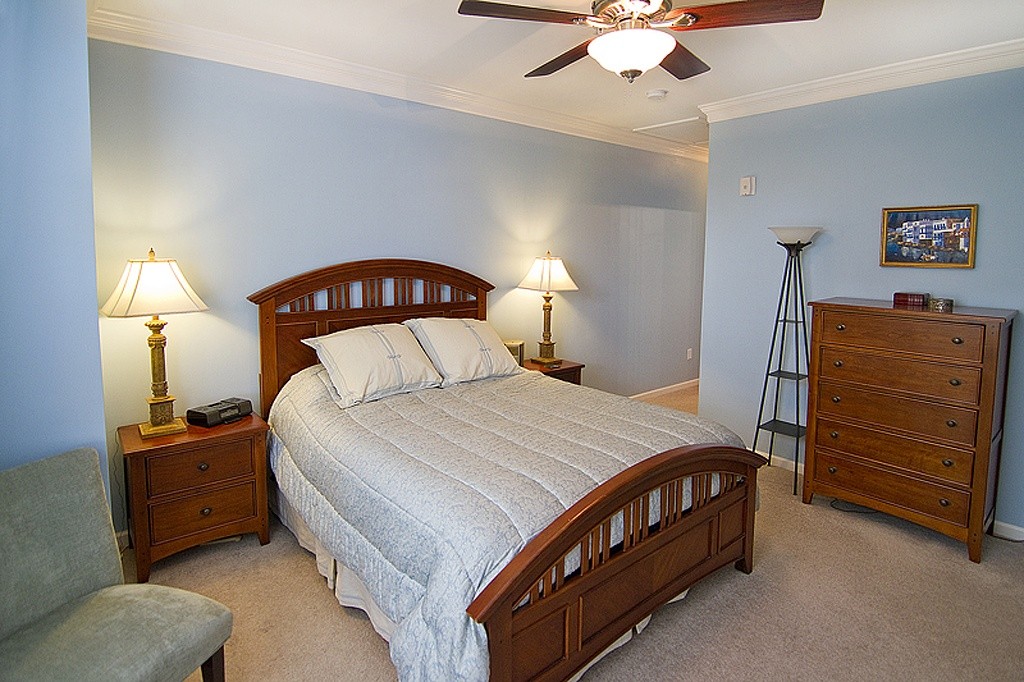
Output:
[520,358,586,386]
[116,409,272,585]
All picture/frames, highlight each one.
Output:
[881,205,979,269]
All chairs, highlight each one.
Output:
[1,448,235,682]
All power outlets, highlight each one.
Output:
[740,177,758,197]
[687,348,693,361]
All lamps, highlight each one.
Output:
[100,247,210,440]
[516,251,580,367]
[570,1,703,83]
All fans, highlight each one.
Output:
[458,1,825,83]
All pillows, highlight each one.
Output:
[402,316,526,389]
[300,324,446,410]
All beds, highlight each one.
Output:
[247,261,773,681]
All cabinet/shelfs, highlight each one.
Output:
[804,297,1022,563]
[749,226,824,499]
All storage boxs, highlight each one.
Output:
[928,299,954,309]
[892,291,931,307]
